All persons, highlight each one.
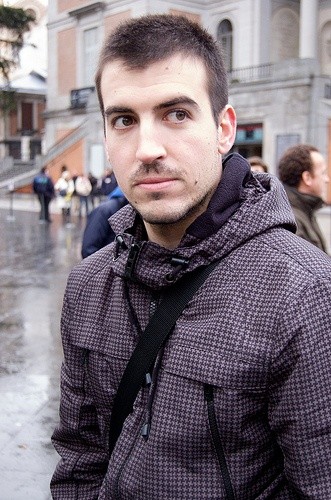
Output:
[31,144,330,260]
[50,13,331,500]
